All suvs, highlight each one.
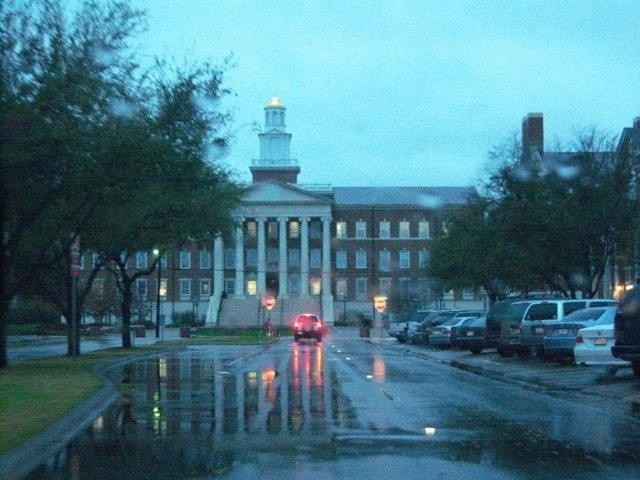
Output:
[295,313,322,341]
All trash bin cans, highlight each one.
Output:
[129,324,135,347]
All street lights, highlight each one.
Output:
[153,248,161,338]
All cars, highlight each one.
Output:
[388,285,640,377]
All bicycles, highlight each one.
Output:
[259,328,281,341]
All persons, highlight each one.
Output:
[265,319,273,336]
